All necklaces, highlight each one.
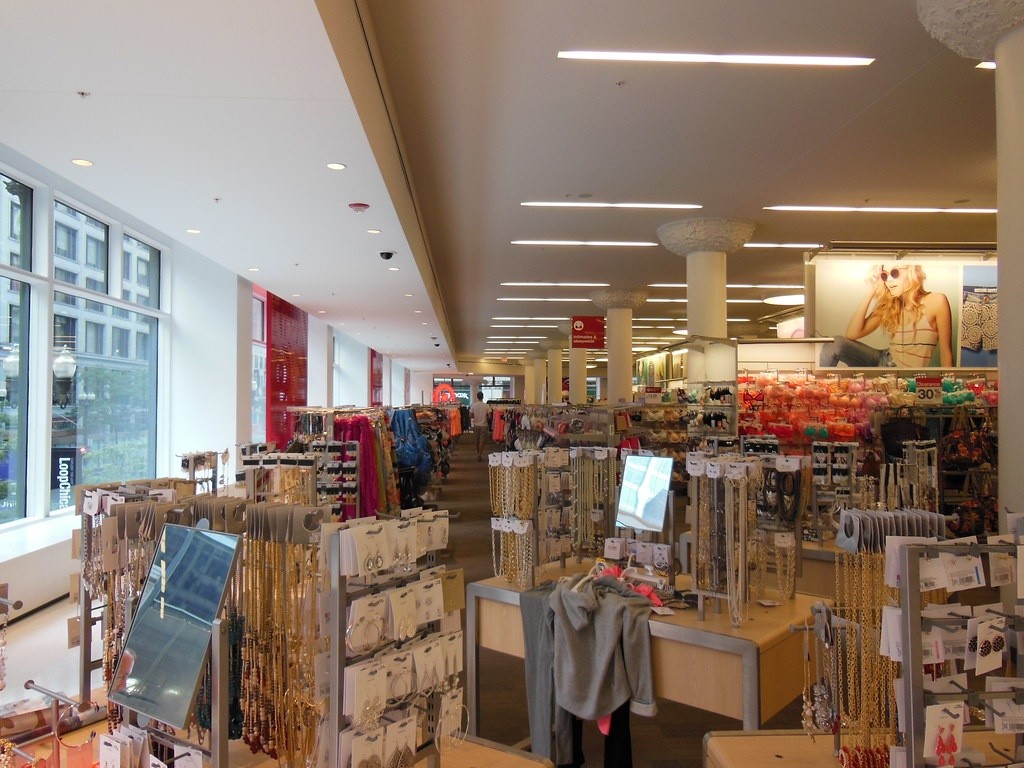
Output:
[83,509,328,768]
[488,457,616,588]
[834,546,899,768]
[859,451,930,512]
[690,468,811,628]
[279,468,311,506]
[801,630,836,743]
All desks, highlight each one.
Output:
[0,680,556,768]
[701,725,1024,768]
[466,554,837,752]
[679,519,948,631]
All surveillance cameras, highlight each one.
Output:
[447,364,450,366]
[466,375,468,376]
[434,344,439,347]
[380,252,393,259]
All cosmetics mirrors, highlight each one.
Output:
[108,524,238,729]
[615,455,673,532]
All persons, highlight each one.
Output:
[469,391,492,460]
[819,262,953,367]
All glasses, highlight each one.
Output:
[673,589,709,605]
[881,268,908,281]
[663,600,698,609]
